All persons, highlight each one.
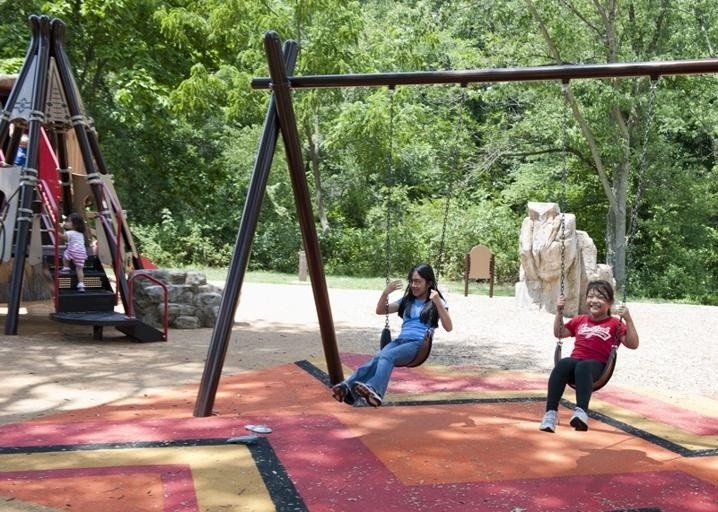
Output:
[47,210,88,293]
[332,262,453,408]
[539,278,639,434]
[9,122,28,165]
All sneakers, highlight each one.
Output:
[538,410,560,433]
[352,382,382,407]
[331,382,349,403]
[568,407,589,432]
[59,266,86,293]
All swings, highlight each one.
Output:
[380,83,467,369]
[556,76,658,392]
